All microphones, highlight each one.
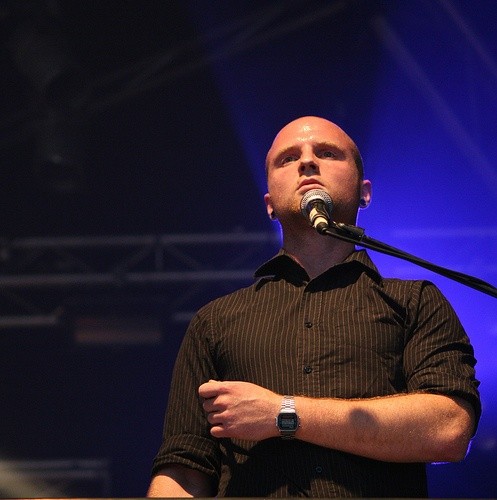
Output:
[300,189,336,235]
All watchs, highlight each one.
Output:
[274,394,301,441]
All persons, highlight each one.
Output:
[146,115,482,500]
